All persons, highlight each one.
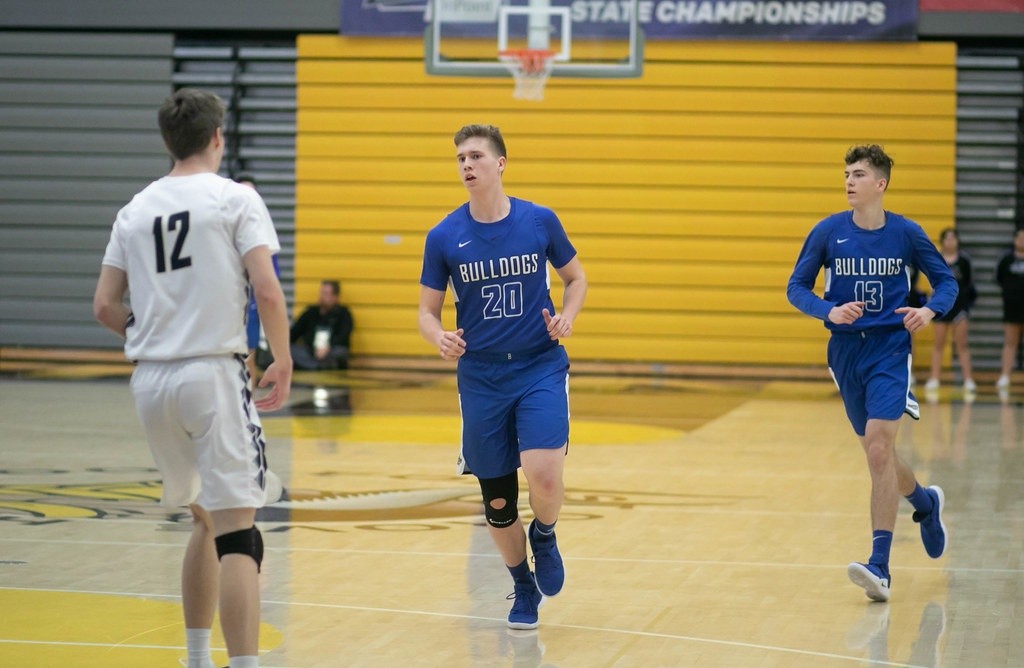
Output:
[925,223,978,388]
[785,144,961,605]
[288,279,356,371]
[92,86,294,668]
[417,124,590,629]
[993,228,1024,387]
[924,389,1024,543]
[847,605,947,668]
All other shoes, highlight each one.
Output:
[960,380,977,391]
[996,376,1010,388]
[925,379,939,390]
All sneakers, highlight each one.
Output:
[506,571,544,630]
[912,485,948,560]
[848,553,891,602]
[528,518,565,598]
